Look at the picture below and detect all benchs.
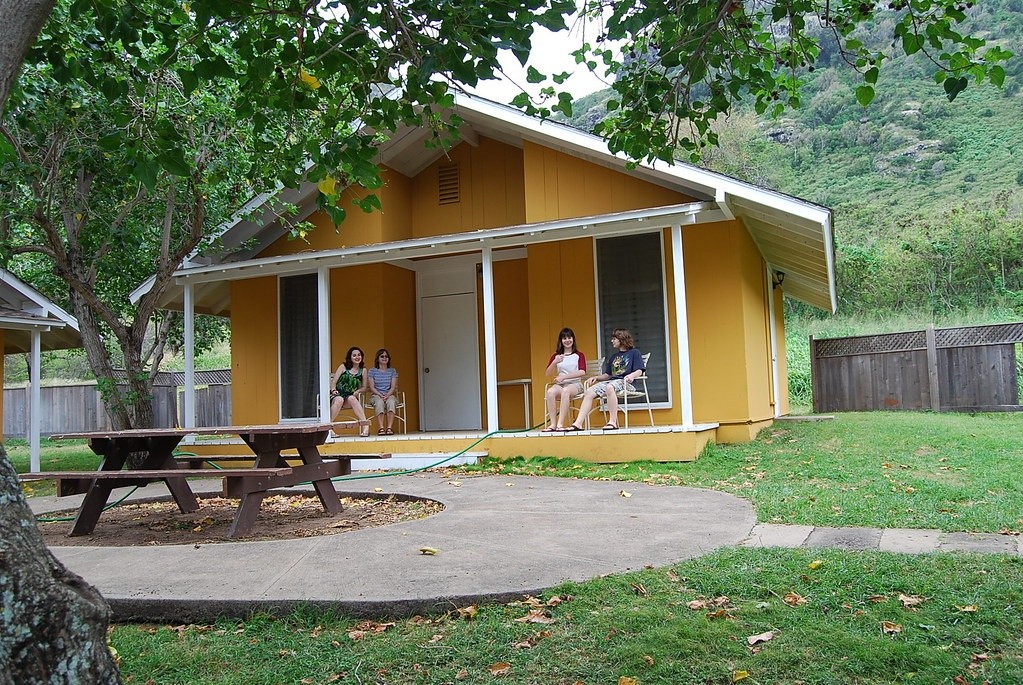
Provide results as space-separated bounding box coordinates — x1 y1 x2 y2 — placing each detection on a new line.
14 466 293 484
171 452 392 463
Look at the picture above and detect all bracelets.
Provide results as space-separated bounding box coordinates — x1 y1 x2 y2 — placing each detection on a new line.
332 390 335 393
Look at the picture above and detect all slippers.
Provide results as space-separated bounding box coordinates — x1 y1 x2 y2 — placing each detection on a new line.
360 432 369 436
555 427 564 432
331 433 339 438
565 425 584 431
542 428 555 432
603 424 619 430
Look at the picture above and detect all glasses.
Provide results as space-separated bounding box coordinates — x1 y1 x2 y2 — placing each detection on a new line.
380 355 389 358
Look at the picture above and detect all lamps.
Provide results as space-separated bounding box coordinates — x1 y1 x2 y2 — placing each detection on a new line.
772 271 785 289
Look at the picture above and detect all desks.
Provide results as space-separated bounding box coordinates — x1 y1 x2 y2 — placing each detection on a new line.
51 419 372 539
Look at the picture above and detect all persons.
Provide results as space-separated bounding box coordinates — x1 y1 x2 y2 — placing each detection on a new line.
368 349 398 436
542 327 586 431
564 328 646 431
330 347 370 438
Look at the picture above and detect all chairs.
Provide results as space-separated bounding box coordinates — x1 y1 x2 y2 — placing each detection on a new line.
585 351 652 429
364 371 407 435
316 373 363 436
543 355 606 430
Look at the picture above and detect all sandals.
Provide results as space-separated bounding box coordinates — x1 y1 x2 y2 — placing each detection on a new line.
386 428 393 435
378 429 385 435
